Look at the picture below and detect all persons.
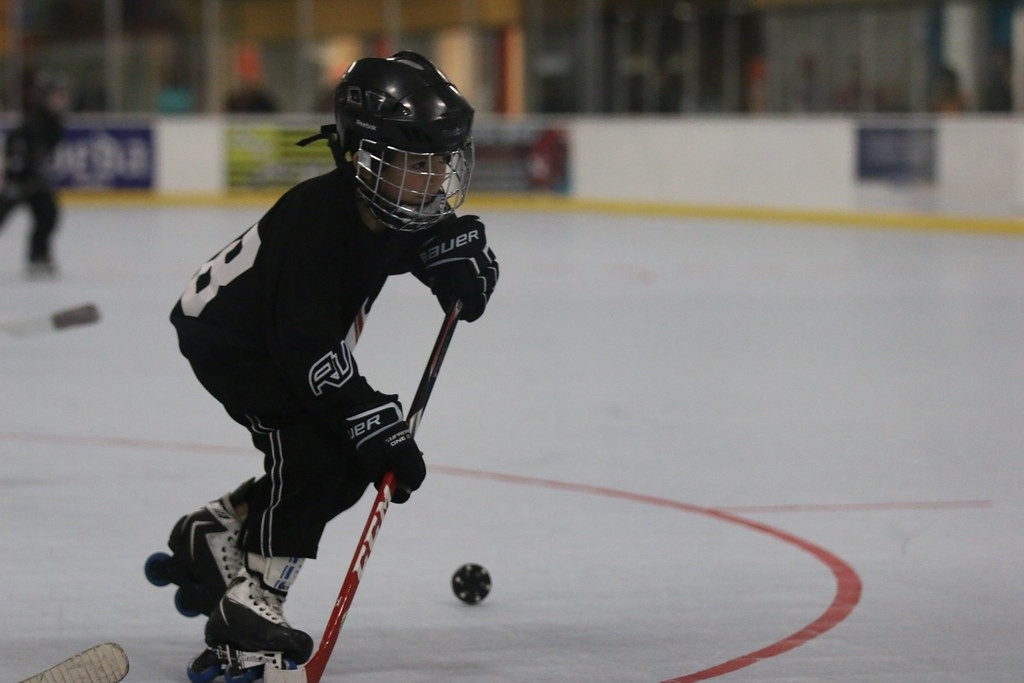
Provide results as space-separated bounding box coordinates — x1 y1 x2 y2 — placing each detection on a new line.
0 78 69 276
146 51 499 683
931 45 1014 113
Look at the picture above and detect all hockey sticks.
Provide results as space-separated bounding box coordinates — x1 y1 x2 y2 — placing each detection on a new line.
1 302 102 339
186 296 464 683
18 641 131 683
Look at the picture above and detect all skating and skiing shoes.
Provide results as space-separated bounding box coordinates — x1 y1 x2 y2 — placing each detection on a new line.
144 475 258 619
185 553 313 682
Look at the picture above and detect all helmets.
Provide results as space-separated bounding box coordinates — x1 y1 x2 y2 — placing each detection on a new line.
296 49 474 234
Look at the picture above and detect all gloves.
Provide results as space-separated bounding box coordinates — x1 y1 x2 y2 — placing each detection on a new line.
343 391 427 504
414 215 499 322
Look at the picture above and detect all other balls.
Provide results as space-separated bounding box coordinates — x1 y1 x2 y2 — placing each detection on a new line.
450 562 492 606
